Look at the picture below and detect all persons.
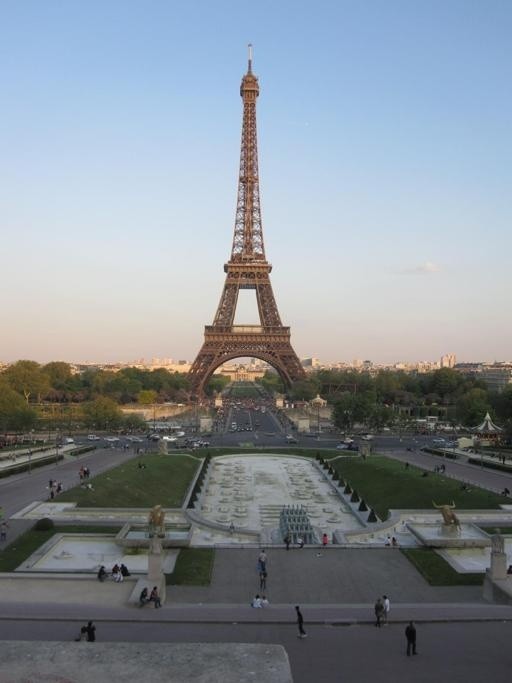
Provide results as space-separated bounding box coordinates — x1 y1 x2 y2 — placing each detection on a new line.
392 537 397 545
384 537 390 545
251 532 304 608
405 621 418 656
295 606 307 638
375 599 384 627
322 534 328 546
0 464 162 642
383 595 390 625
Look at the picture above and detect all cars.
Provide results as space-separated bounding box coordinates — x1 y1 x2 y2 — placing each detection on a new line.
87 430 211 449
287 435 299 444
336 434 360 451
